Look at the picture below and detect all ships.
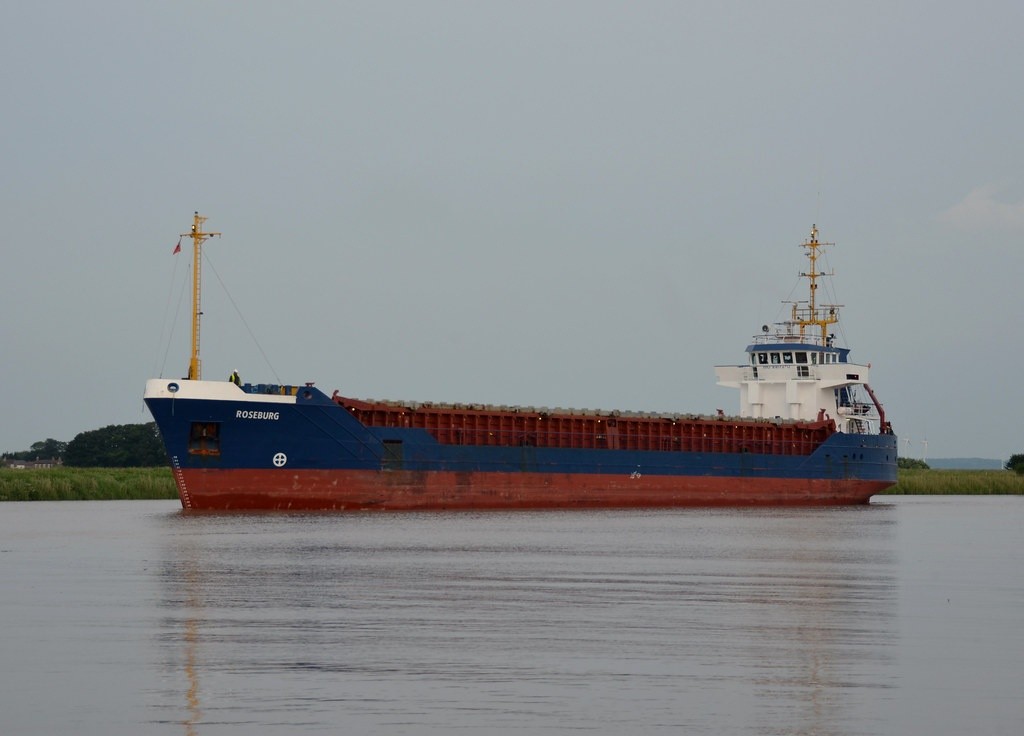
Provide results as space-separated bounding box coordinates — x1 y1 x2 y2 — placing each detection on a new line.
141 210 902 513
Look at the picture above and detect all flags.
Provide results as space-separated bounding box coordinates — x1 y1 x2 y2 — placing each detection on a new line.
172 241 181 255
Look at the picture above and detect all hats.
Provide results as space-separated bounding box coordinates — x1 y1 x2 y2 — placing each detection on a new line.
234 370 238 373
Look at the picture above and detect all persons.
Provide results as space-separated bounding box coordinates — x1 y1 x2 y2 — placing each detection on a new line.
229 369 241 387
